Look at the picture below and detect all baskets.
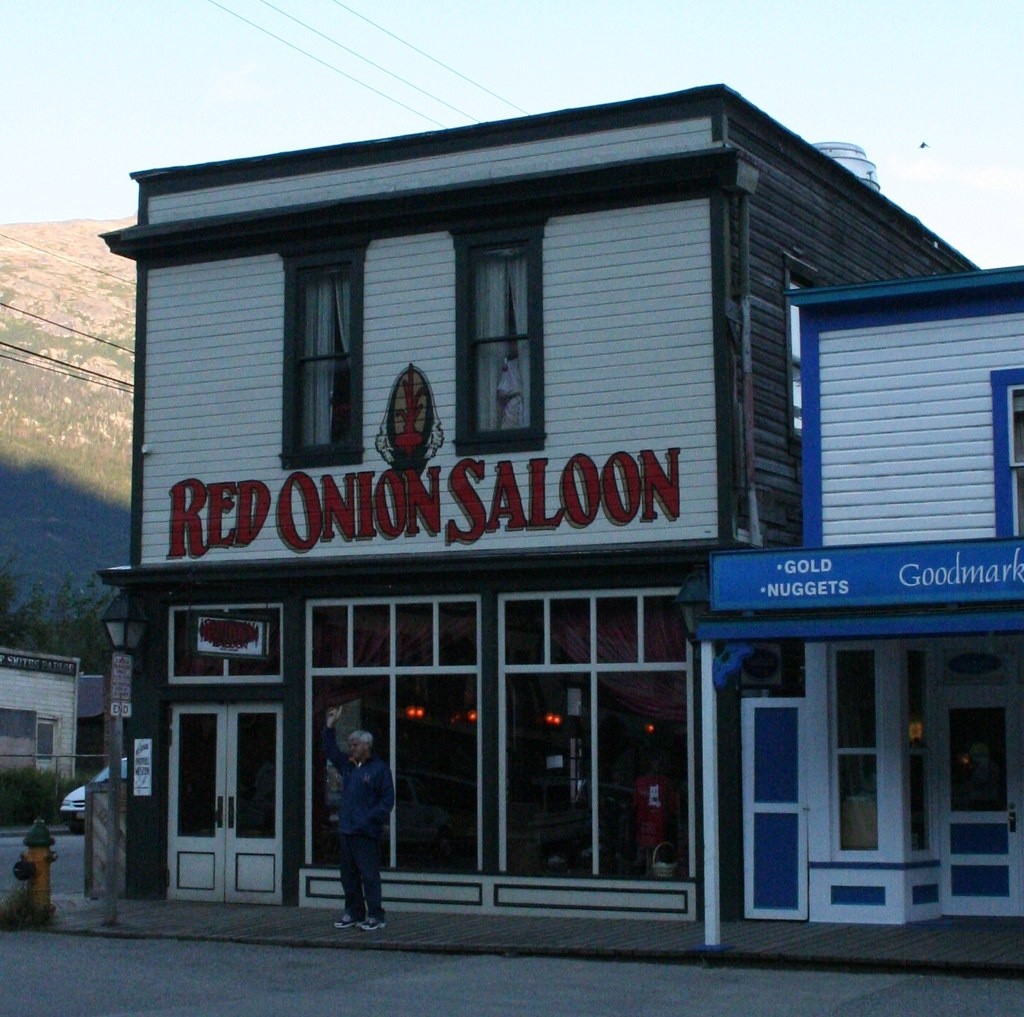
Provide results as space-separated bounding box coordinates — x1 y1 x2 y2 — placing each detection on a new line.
651 842 679 879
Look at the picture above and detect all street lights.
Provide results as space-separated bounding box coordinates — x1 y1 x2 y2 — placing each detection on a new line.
97 588 154 925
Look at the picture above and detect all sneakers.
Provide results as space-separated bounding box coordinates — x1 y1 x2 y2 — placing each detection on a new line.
360 917 385 930
334 914 368 928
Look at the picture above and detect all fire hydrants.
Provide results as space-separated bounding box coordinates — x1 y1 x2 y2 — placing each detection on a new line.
13 814 59 926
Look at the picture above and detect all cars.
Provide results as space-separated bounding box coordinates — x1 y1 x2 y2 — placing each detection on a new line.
58 756 128 835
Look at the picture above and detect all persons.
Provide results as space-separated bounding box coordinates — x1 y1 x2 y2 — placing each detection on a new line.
497 358 522 429
322 707 394 930
